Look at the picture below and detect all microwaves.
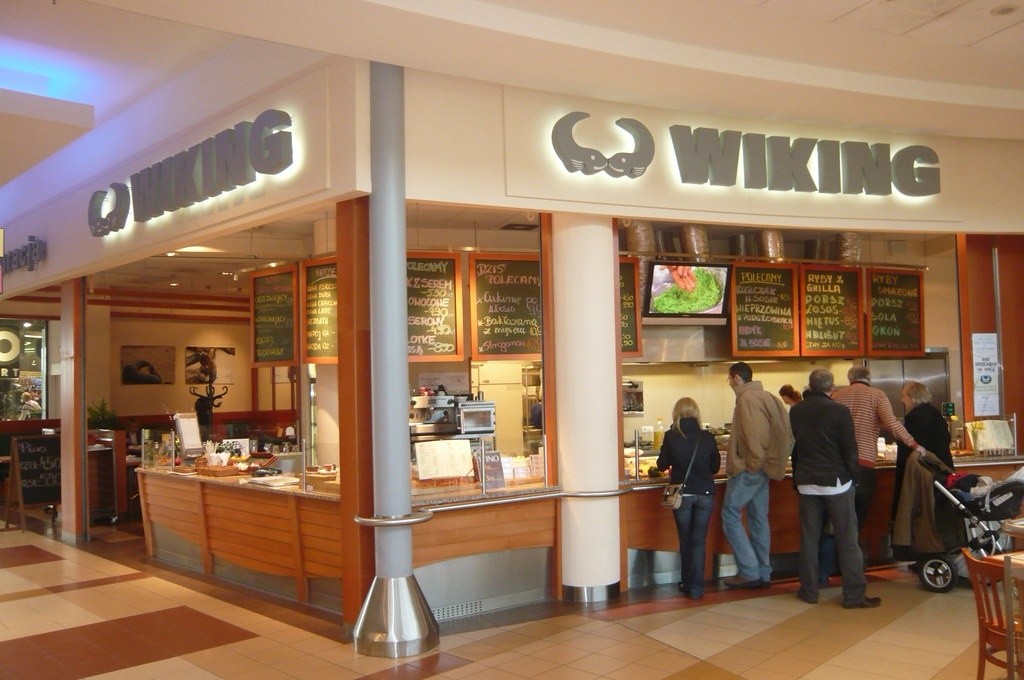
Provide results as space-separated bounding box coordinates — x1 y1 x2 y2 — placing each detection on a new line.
455 408 496 434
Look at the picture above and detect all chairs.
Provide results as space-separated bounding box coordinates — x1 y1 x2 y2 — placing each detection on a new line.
960 546 1024 680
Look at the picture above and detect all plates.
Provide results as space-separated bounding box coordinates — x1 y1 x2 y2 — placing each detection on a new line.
248 476 300 486
624 448 644 457
651 268 725 314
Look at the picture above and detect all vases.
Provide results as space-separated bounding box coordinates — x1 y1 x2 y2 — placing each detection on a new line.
969 431 981 456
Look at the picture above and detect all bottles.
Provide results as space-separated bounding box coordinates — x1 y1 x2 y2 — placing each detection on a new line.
216 441 242 457
264 442 298 453
154 442 160 459
437 384 446 396
163 438 177 458
252 443 256 452
654 417 663 451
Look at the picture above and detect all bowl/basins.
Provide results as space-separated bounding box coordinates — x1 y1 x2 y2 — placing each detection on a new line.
455 397 468 402
99 438 113 447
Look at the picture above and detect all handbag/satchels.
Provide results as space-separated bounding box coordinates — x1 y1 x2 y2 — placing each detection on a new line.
660 482 686 510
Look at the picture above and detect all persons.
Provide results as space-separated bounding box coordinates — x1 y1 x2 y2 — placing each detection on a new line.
529 393 543 455
656 397 721 600
818 365 926 587
797 369 882 609
125 417 143 457
894 379 956 571
780 383 802 406
660 265 697 293
722 362 793 588
19 390 41 420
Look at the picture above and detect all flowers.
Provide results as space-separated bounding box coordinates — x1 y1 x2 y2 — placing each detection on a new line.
950 414 984 446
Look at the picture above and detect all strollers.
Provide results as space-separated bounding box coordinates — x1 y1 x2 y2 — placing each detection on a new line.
911 448 1024 594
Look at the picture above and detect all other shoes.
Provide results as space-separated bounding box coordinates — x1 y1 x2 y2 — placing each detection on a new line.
679 581 699 599
845 595 881 607
725 574 771 588
798 589 816 604
908 562 917 568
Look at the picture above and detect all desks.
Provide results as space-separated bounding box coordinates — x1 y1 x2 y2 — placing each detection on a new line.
126 445 140 518
982 551 1024 619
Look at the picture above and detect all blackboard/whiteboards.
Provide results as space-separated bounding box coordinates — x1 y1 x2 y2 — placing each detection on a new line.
799 264 864 357
298 255 339 364
730 261 800 357
406 250 464 362
469 253 543 360
249 264 298 368
476 452 506 489
866 268 925 357
7 435 61 508
619 255 643 359
